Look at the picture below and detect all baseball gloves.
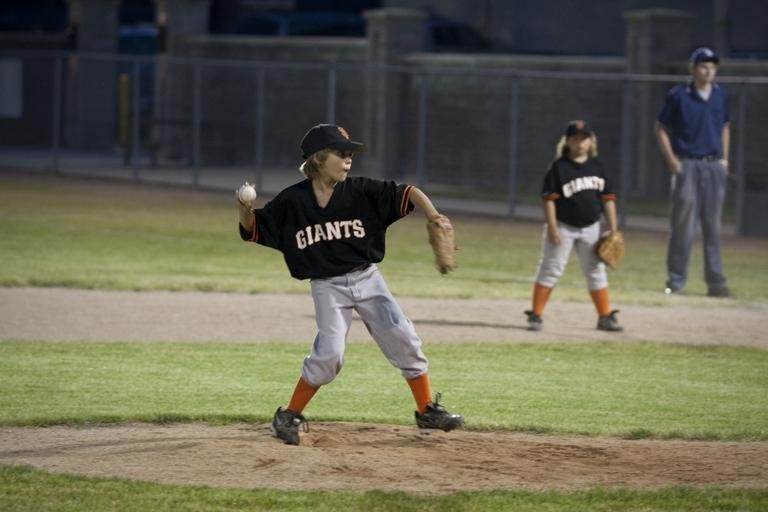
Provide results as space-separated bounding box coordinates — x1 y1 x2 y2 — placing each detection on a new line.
596 230 624 269
428 214 456 274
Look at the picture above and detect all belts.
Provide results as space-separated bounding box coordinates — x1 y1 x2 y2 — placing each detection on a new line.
683 155 717 160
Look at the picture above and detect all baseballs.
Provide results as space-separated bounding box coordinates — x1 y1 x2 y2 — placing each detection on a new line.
238 185 256 204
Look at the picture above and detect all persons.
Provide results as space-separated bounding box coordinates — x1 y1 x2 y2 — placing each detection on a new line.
231 121 465 447
651 46 737 299
521 118 630 333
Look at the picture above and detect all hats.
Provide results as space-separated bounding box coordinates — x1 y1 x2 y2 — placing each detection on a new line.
300 124 367 159
692 48 719 64
567 121 590 137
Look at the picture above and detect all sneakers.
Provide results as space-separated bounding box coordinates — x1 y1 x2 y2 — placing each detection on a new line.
707 285 731 297
597 309 623 331
526 310 542 329
274 407 305 445
665 283 682 295
414 401 464 430
